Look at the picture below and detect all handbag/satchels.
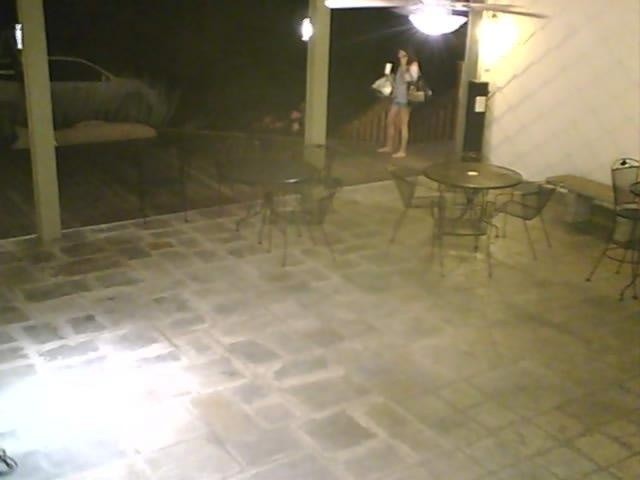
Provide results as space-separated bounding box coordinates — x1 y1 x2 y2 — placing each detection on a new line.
371 73 394 97
407 76 432 103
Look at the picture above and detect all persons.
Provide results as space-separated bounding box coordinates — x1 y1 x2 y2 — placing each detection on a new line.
377 48 420 158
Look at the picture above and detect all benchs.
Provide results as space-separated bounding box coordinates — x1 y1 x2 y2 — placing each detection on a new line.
546 174 640 242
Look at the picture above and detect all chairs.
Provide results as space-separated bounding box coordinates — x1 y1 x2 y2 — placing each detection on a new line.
221 143 343 268
389 160 557 278
139 135 190 225
584 158 640 303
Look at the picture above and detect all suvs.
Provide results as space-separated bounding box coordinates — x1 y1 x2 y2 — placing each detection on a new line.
1 56 169 125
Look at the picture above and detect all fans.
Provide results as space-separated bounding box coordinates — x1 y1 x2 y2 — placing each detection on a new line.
324 1 544 19
